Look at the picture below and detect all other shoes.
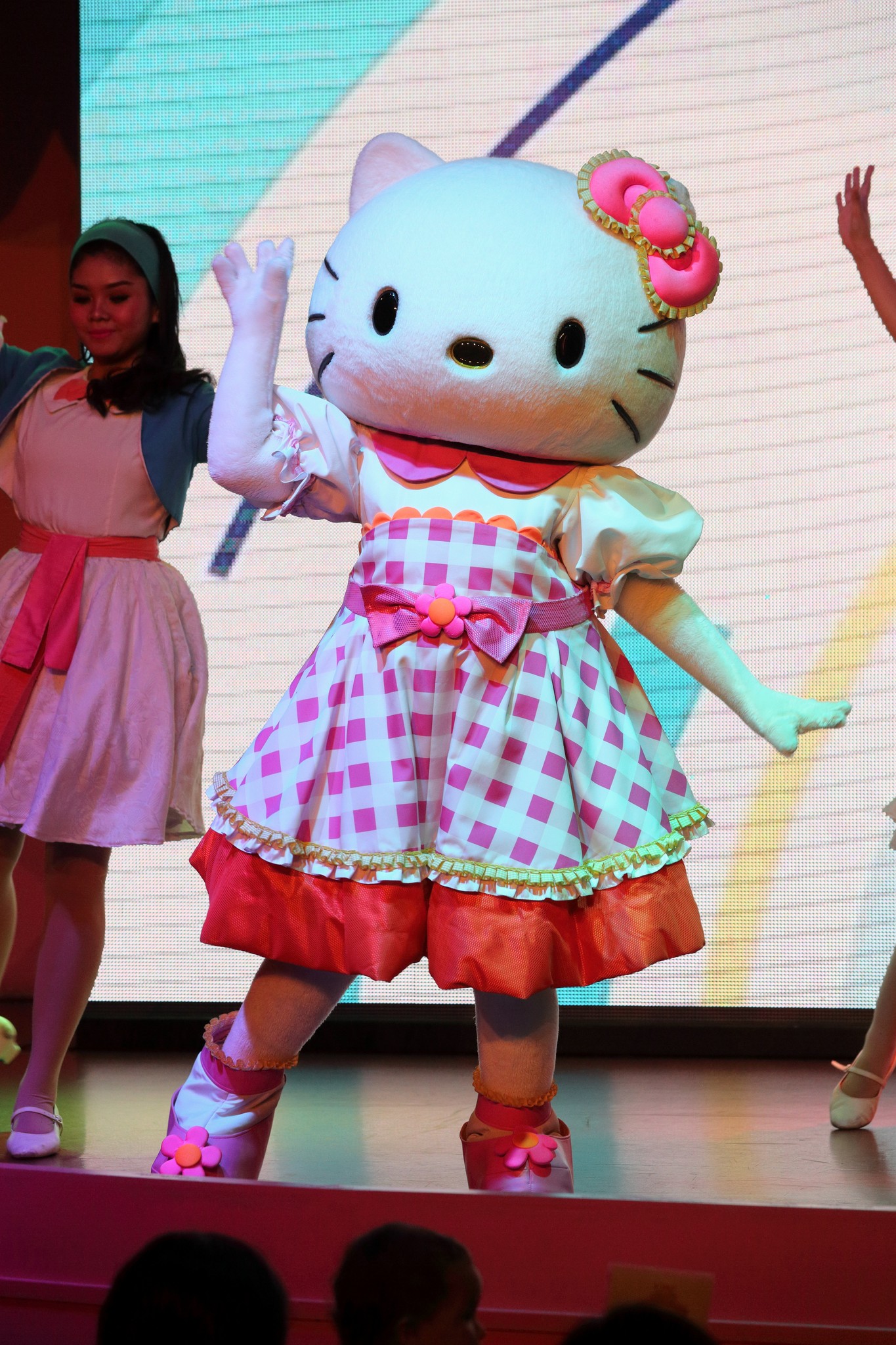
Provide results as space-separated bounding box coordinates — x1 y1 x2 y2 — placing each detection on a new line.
826 1048 896 1131
7 1101 64 1163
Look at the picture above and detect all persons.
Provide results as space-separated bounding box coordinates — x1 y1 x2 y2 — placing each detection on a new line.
566 1302 713 1345
330 1219 483 1345
0 219 215 1150
831 162 896 1131
92 1227 291 1345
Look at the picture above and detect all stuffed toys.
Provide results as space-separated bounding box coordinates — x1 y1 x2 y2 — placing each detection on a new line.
150 133 852 1199
